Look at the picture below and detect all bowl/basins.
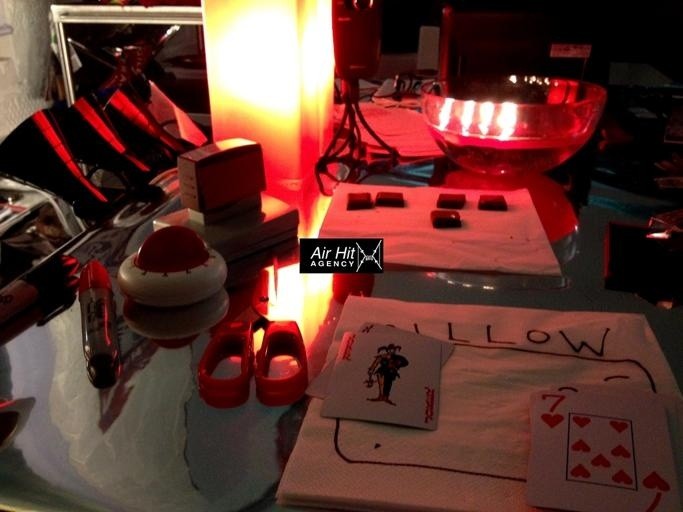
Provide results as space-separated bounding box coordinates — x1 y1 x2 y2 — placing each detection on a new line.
422 74 608 177
